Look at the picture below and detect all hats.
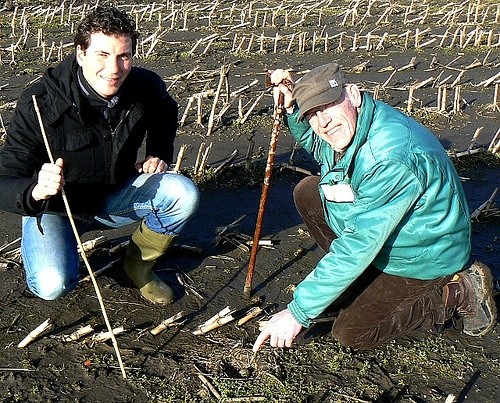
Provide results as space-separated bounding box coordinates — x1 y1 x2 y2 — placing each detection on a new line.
291 63 346 124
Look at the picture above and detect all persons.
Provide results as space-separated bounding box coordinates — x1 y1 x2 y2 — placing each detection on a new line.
252 64 498 353
0 6 200 307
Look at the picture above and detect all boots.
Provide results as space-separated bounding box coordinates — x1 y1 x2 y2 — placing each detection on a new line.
123 218 175 306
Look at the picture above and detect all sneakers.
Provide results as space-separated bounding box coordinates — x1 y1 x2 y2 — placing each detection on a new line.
438 261 497 336
